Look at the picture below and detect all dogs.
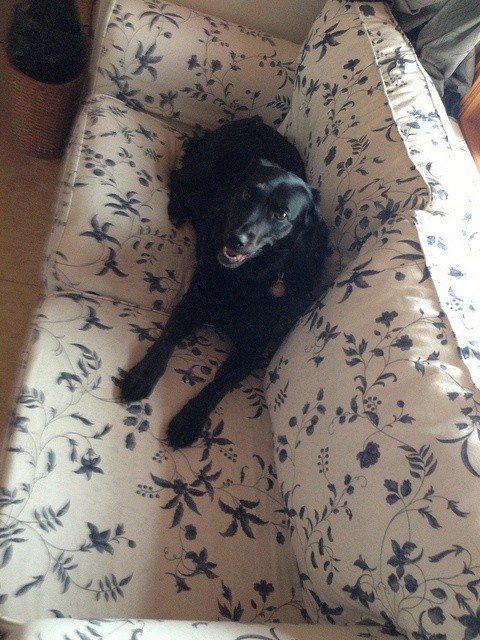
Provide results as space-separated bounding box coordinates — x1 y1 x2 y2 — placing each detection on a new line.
120 118 329 450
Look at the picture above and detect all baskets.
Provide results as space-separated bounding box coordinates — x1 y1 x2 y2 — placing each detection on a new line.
8 57 85 160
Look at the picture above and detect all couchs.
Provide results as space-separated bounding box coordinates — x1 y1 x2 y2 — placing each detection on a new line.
0 1 479 640
